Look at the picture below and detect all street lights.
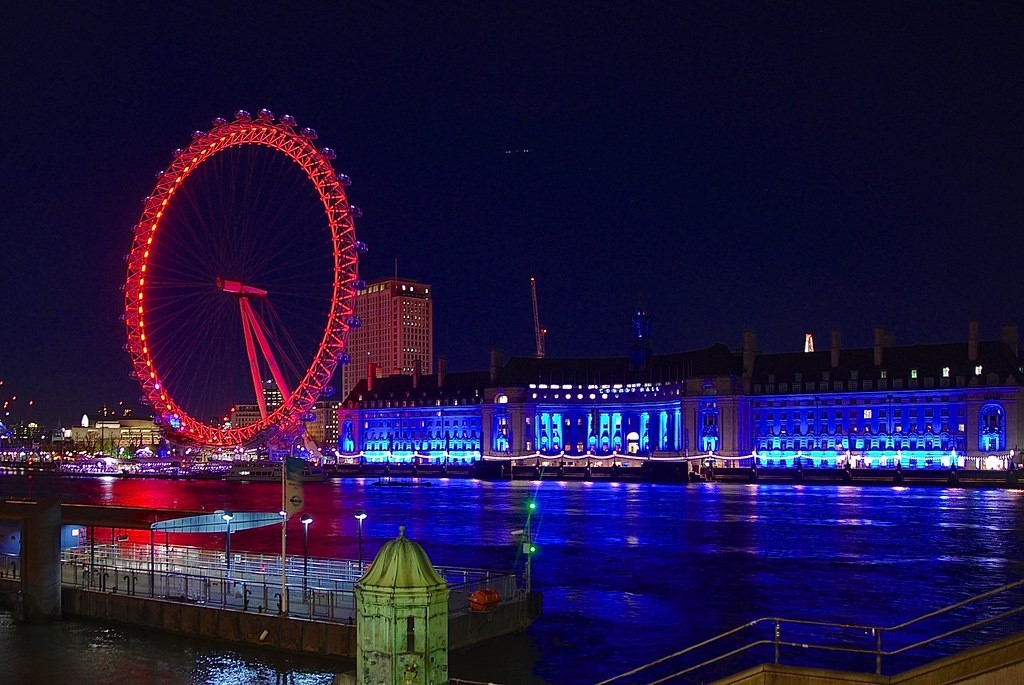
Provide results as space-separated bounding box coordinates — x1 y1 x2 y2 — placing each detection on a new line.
536 451 540 466
586 451 591 473
612 451 618 467
560 450 564 468
355 511 367 573
222 513 233 593
301 514 312 600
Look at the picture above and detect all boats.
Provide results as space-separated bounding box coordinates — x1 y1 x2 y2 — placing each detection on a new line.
221 460 326 482
373 475 431 486
